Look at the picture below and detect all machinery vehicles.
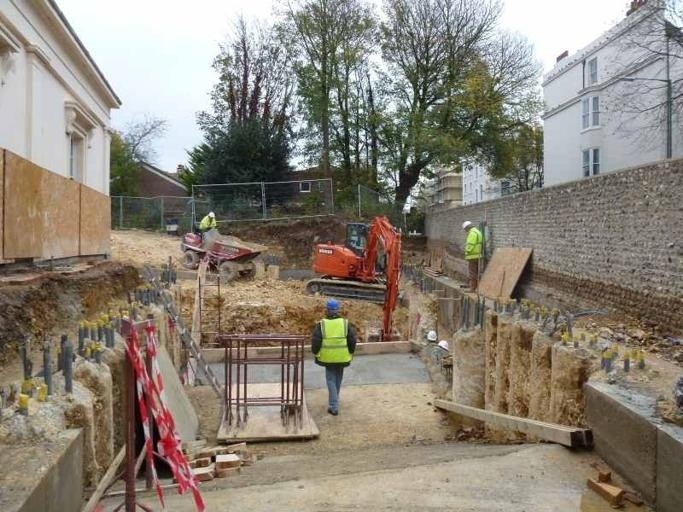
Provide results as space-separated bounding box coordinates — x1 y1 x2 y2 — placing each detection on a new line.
304 215 403 342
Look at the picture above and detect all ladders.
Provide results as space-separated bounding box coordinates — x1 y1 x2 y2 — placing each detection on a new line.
149 277 225 398
199 276 220 348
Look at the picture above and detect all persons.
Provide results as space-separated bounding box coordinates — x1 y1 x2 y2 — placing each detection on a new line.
456 220 486 295
311 296 356 416
196 212 218 232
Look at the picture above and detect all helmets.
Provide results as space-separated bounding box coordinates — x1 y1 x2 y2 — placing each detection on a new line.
462 220 471 230
326 300 338 310
209 211 215 218
438 340 449 351
427 330 437 341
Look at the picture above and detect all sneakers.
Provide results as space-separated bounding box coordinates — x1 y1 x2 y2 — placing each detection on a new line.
328 408 338 416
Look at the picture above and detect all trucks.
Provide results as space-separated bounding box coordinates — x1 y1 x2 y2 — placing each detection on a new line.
181 230 268 282
